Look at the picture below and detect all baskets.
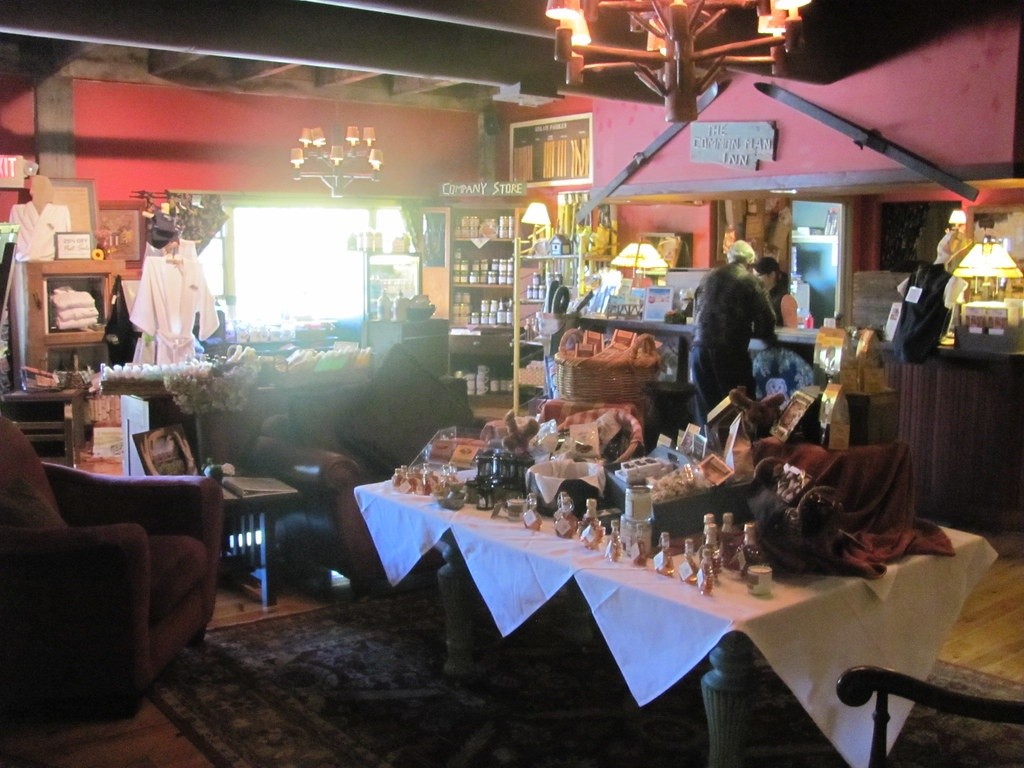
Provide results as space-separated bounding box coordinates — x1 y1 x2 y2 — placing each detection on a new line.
555 352 656 404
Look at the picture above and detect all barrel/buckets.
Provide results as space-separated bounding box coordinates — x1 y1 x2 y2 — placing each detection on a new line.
530 462 601 520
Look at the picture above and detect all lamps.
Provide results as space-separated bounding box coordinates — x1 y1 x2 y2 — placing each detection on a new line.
545 0 812 123
520 203 551 258
610 242 669 289
952 244 1024 335
290 99 384 197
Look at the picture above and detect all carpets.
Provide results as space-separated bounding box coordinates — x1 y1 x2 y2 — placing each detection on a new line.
141 599 1024 768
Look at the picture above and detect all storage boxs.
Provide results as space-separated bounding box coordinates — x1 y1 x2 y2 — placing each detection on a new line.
554 327 660 404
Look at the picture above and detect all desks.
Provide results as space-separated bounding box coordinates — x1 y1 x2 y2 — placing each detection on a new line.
219 476 302 607
353 479 1000 768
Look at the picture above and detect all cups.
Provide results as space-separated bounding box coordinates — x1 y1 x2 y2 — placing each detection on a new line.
462 365 489 396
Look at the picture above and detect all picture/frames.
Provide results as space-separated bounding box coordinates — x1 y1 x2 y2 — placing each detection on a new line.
53 232 94 260
47 177 97 258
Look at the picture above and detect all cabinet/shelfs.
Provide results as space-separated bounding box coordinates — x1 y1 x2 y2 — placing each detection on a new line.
443 201 521 417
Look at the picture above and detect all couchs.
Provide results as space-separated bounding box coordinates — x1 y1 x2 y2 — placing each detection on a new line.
0 414 225 716
251 353 488 598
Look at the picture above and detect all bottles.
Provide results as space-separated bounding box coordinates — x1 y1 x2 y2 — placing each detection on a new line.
655 531 674 575
204 346 255 376
100 362 213 385
679 513 772 598
622 487 652 567
466 454 536 511
525 493 542 531
490 377 514 395
527 271 562 300
376 290 391 319
366 232 383 256
578 498 623 563
452 291 514 326
259 350 275 382
790 280 814 332
392 461 459 496
455 215 515 239
525 312 543 342
554 492 577 540
453 258 514 284
394 291 411 319
288 346 371 371
824 207 839 235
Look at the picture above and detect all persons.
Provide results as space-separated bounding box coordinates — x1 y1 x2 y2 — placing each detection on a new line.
687 239 777 413
752 257 788 328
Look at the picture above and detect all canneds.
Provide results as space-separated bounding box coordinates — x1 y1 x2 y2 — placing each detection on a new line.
453 216 516 326
526 272 563 300
